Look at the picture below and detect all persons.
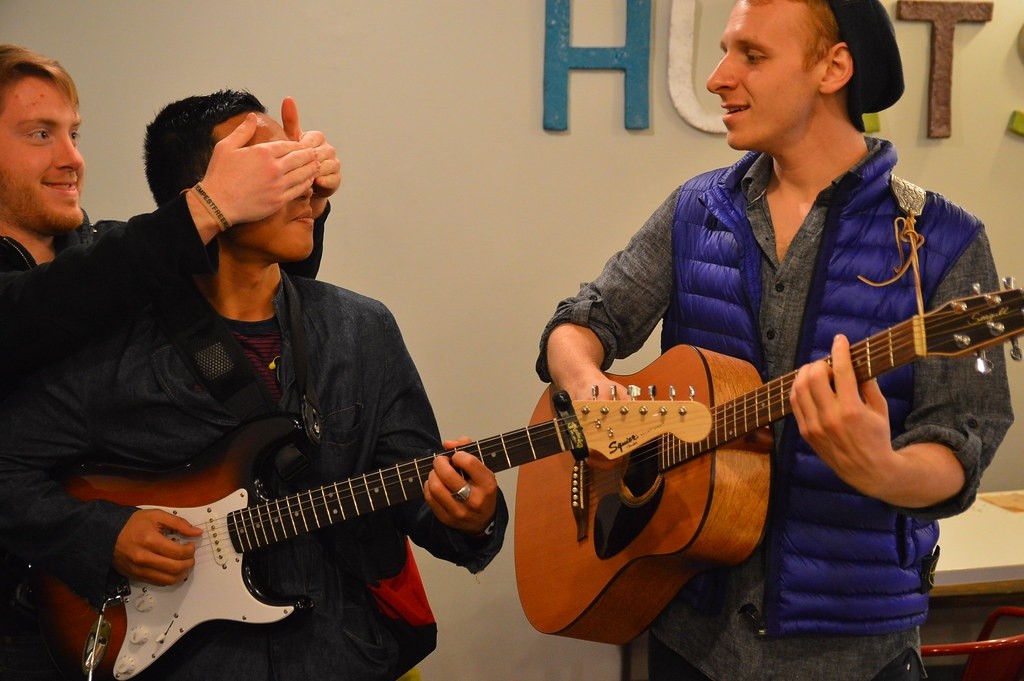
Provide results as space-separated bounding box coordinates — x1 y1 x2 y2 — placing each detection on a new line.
0 47 340 382
534 0 1013 681
0 91 509 681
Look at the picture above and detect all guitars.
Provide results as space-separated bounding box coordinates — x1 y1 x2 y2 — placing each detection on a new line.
34 385 713 681
514 276 1024 646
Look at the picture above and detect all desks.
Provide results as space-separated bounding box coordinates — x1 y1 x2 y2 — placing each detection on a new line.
928 490 1024 596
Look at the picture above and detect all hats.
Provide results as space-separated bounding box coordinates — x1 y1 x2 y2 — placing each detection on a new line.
830 0 904 132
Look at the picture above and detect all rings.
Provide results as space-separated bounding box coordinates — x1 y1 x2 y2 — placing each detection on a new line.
453 484 472 501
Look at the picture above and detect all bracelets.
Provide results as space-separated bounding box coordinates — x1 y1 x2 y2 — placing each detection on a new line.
191 181 232 233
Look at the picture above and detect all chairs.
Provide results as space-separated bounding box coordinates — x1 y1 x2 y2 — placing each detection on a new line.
920 606 1024 681
